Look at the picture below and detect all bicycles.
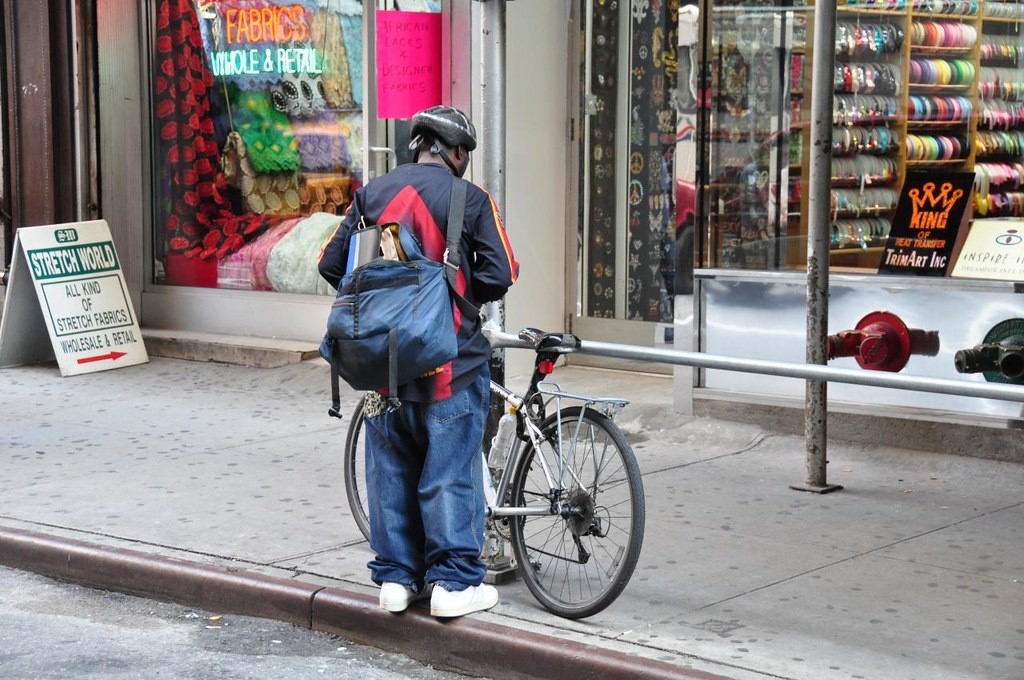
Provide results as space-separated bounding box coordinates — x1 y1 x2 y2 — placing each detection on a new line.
343 327 646 620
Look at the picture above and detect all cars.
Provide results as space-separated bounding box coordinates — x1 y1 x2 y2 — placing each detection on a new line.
675 124 804 294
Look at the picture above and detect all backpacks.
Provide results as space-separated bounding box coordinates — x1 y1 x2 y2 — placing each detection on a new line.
316 175 482 420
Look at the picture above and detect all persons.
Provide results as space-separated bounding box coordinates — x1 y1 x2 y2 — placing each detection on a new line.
318 106 520 619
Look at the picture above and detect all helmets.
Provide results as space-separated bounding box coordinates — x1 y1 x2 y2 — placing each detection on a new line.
408 103 478 177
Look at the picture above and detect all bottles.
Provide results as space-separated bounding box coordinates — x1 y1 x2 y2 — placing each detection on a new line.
488 405 517 470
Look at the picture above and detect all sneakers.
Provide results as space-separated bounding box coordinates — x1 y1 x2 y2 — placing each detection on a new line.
380 581 433 612
430 583 499 617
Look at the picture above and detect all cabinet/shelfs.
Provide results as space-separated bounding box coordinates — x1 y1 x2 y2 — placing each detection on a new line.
708 1 1024 267
